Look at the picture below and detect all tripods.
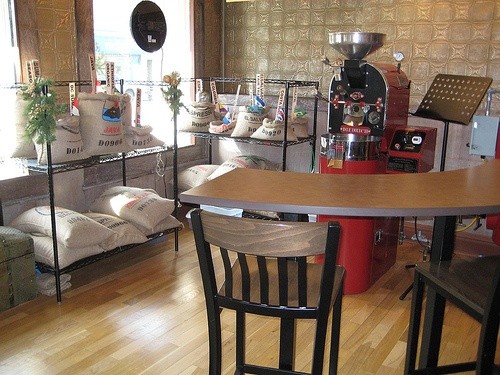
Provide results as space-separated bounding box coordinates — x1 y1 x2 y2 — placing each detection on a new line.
399 74 493 302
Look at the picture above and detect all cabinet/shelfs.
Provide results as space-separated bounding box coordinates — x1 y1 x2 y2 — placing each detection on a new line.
183 77 319 173
6 80 182 303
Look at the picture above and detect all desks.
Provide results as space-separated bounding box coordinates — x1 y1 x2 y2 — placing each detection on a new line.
179 157 500 375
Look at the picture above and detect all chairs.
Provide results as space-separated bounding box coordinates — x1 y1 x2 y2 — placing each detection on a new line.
403 254 500 375
190 208 347 375
